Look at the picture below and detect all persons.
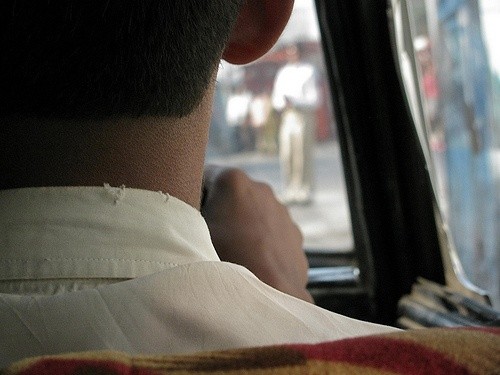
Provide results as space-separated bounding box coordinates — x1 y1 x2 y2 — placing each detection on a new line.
0 0 411 375
271 40 326 207
